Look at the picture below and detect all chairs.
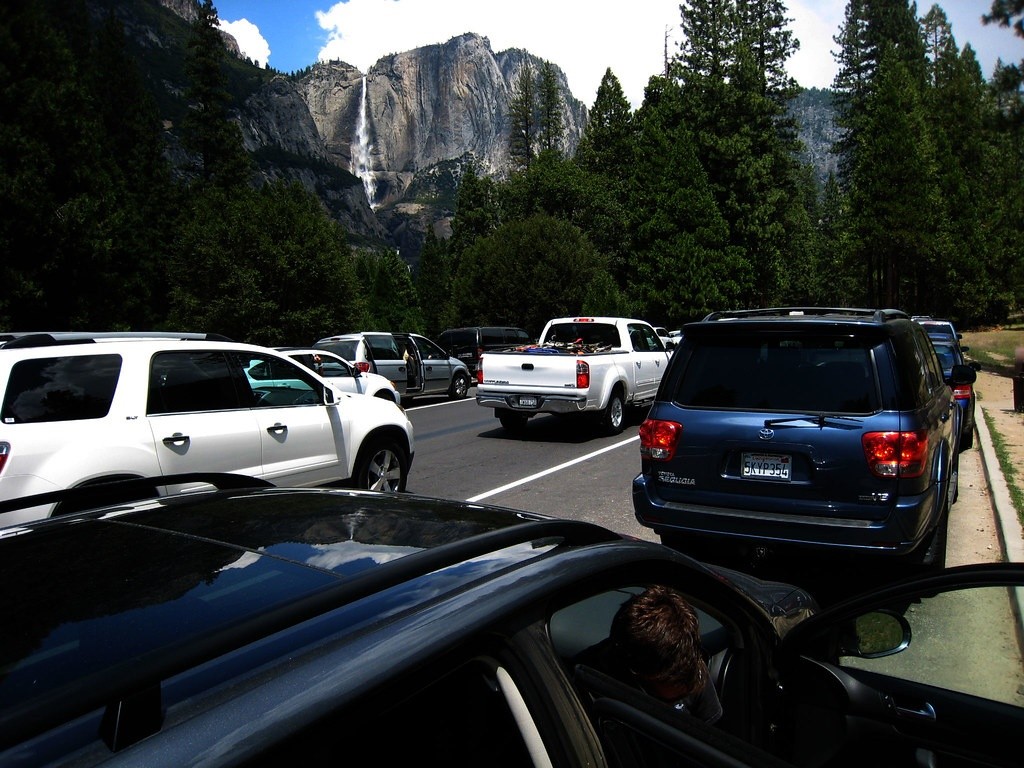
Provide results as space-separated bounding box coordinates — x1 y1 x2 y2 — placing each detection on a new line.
818 358 875 410
164 368 199 409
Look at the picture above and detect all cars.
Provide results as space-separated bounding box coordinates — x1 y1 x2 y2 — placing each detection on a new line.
909 315 980 446
633 305 977 568
0 330 416 542
653 326 674 350
0 472 1024 768
668 331 681 339
244 346 402 406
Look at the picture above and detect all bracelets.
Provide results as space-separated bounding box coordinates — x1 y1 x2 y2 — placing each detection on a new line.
318 364 323 367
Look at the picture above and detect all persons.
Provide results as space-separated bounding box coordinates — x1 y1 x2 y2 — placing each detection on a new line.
309 354 324 375
573 586 722 726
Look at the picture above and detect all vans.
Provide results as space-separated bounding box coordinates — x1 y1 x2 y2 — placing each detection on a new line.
311 332 472 401
436 326 534 376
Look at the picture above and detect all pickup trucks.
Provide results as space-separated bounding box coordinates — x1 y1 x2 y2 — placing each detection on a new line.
476 315 674 430
